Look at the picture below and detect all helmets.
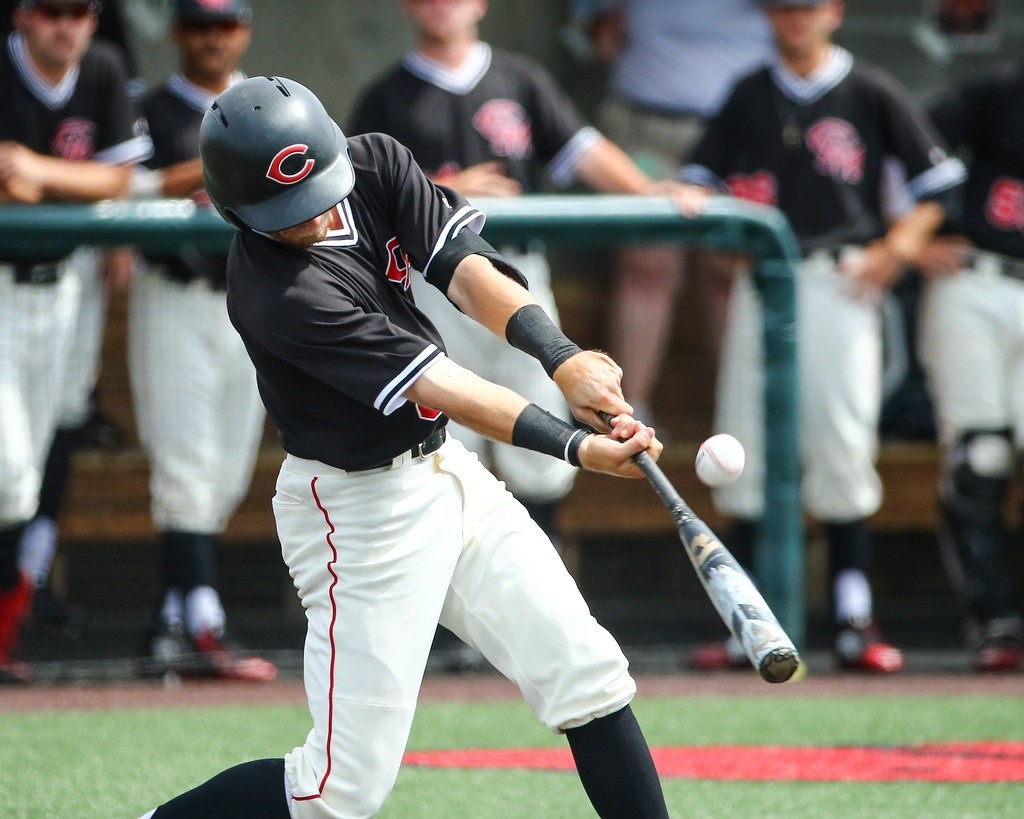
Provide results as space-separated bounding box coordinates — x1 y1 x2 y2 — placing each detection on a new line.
199 75 355 233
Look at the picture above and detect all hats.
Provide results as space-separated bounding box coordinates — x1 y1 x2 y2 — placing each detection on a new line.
177 0 250 27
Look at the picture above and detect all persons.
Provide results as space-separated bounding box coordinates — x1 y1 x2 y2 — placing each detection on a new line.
0 0 155 676
670 2 972 676
140 74 674 819
554 0 1024 675
114 0 281 685
342 1 702 675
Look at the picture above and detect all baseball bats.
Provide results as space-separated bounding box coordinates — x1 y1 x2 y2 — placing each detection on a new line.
599 413 801 684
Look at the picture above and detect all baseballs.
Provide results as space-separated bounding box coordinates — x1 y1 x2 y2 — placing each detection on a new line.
694 433 745 488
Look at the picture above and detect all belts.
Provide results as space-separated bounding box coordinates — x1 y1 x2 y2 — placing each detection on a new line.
13 262 61 286
349 425 448 473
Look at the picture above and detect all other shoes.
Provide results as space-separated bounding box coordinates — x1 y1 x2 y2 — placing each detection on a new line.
971 636 1020 669
172 640 279 683
838 627 906 672
688 640 755 669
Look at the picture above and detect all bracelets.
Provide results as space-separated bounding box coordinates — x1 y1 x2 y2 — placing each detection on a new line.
505 304 583 382
512 402 590 470
